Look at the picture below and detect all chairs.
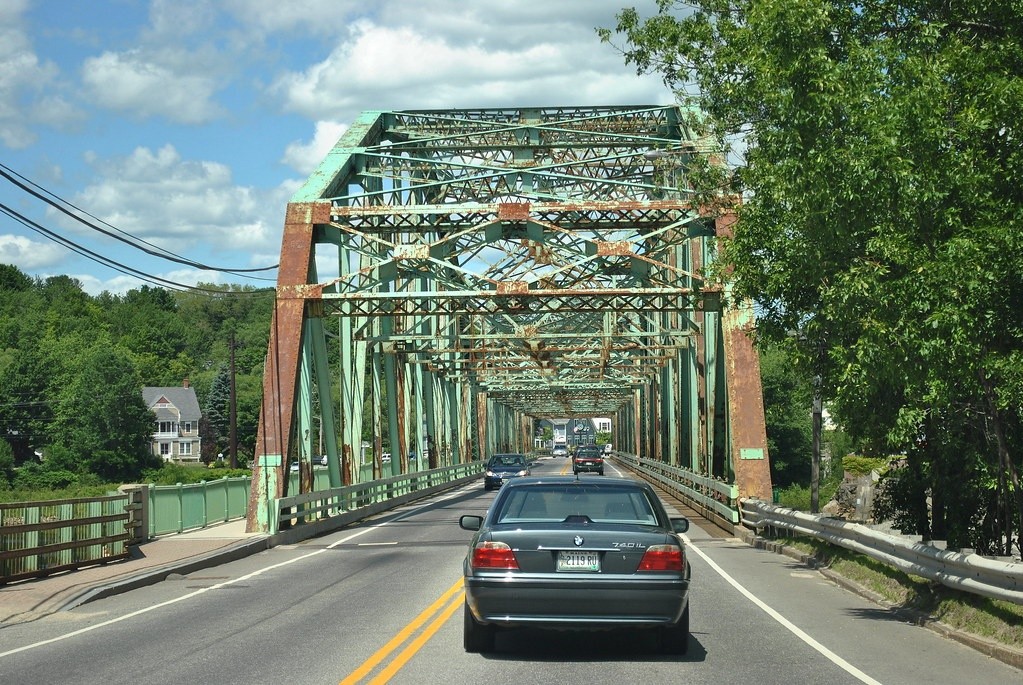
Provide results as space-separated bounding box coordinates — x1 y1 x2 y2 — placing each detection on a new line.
516 502 546 518
606 502 639 518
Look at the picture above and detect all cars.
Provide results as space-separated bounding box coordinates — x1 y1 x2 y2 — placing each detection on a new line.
482 454 533 491
291 461 299 471
571 443 612 476
457 473 693 654
551 443 570 458
